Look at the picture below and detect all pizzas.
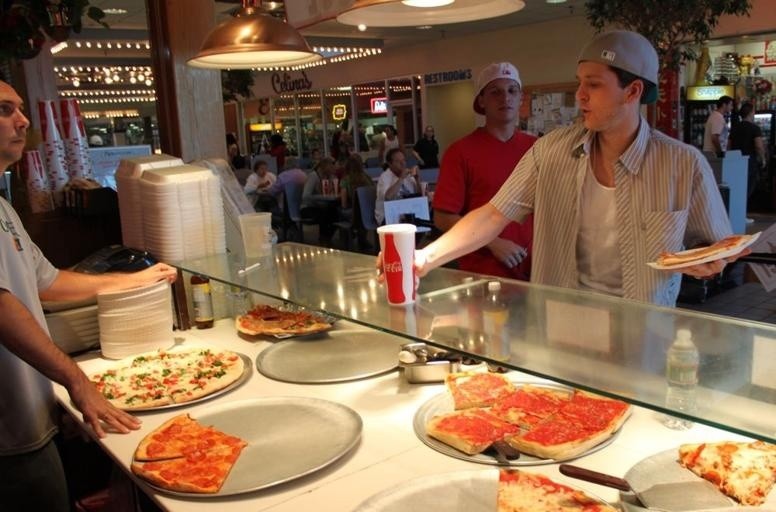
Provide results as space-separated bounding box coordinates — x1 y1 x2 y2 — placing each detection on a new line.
235 304 332 336
88 348 244 410
427 371 630 462
657 235 750 266
497 467 616 512
130 412 247 493
678 440 776 506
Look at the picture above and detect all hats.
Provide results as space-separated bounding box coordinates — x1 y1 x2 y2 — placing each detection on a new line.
472 63 522 115
577 31 658 104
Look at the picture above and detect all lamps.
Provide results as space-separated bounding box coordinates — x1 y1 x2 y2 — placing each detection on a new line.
336 1 526 31
182 0 323 72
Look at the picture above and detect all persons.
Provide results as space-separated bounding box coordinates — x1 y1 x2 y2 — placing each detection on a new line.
431 61 542 282
702 95 732 152
375 29 753 375
728 103 768 223
224 124 444 249
0 80 178 511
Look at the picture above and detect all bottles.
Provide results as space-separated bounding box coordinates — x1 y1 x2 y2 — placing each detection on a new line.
229 261 249 319
456 274 483 338
483 279 513 372
400 184 406 198
693 105 717 118
751 95 776 111
692 133 703 147
662 330 702 431
692 120 704 131
189 260 216 330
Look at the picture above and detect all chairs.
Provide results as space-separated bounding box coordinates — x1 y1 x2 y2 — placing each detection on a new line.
234 147 440 244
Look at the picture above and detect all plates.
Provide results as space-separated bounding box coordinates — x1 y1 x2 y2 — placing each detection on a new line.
46 305 99 355
98 275 174 360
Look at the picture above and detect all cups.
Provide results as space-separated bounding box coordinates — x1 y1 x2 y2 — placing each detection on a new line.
376 223 418 309
322 180 329 195
22 149 52 216
417 182 428 196
39 99 70 208
333 179 339 193
239 210 272 259
57 97 94 180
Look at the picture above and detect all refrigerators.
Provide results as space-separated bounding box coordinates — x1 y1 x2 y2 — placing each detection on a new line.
684 86 745 161
